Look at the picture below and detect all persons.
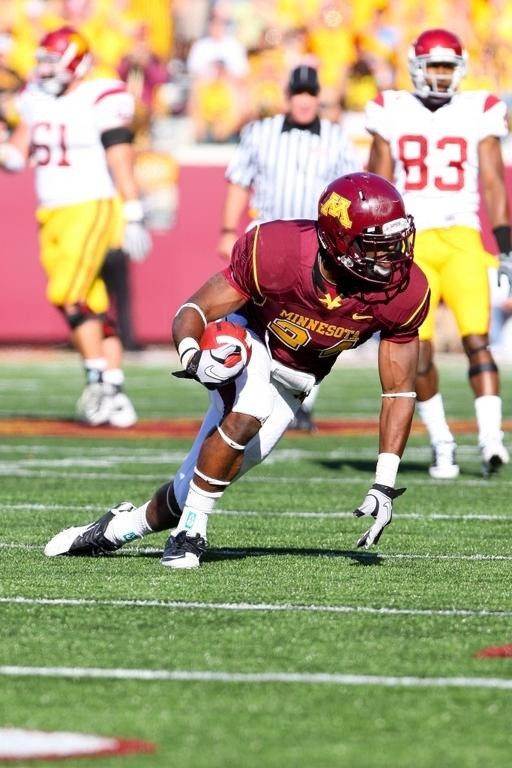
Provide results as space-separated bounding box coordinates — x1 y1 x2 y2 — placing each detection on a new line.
362 28 512 480
220 66 360 432
0 1 512 144
0 25 143 429
43 172 431 568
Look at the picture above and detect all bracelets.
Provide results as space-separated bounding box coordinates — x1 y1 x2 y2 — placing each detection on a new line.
220 227 237 234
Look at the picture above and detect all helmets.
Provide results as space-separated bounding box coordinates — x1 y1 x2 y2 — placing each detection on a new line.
317 172 416 285
33 27 93 96
409 29 468 106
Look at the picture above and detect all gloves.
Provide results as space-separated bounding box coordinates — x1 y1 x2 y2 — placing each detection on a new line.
353 484 407 550
121 221 151 261
498 252 512 296
171 343 246 391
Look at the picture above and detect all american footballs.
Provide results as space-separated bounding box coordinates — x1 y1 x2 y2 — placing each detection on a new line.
201 322 252 369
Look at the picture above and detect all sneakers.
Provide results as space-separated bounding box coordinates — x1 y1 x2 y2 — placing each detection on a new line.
429 441 459 479
479 431 508 468
77 368 138 427
43 502 137 556
161 529 209 568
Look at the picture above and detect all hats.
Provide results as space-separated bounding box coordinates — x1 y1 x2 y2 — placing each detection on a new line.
289 67 317 94
